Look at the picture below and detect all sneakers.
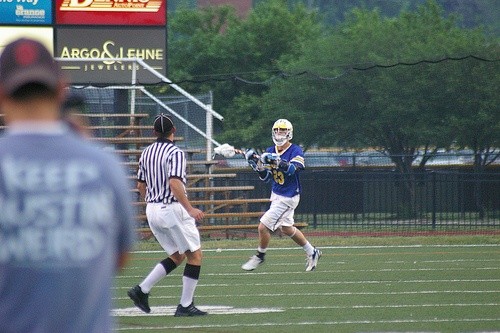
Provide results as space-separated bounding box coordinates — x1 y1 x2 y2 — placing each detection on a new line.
175 303 207 317
128 285 151 313
305 246 323 272
242 255 264 270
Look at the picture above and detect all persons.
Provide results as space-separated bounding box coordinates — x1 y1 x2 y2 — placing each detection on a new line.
1 38 139 333
127 112 208 316
240 118 323 271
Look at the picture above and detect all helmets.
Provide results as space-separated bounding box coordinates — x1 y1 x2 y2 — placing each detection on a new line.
272 119 293 146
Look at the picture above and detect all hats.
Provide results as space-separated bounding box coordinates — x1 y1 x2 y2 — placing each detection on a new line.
152 113 173 133
0 39 57 95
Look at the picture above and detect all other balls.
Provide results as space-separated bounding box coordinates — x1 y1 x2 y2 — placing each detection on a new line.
216 249 221 252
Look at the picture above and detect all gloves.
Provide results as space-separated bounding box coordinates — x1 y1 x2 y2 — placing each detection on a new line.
260 153 280 165
244 147 259 168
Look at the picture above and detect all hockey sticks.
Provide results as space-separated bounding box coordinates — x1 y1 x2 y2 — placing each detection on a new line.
213 143 284 166
